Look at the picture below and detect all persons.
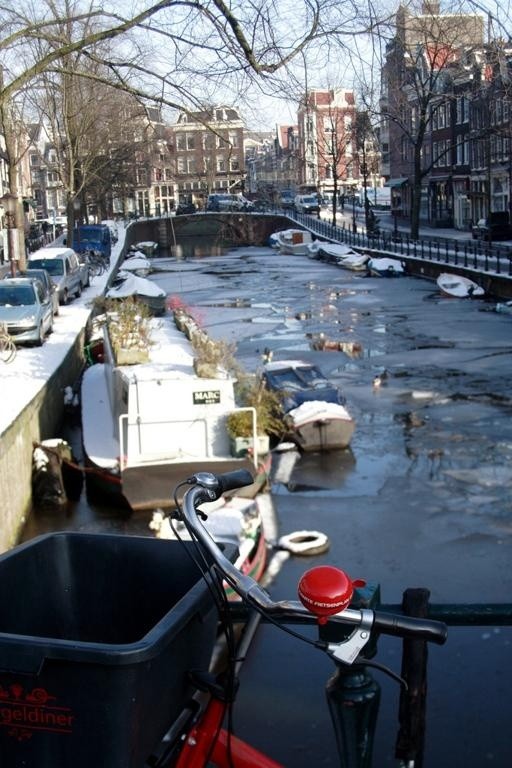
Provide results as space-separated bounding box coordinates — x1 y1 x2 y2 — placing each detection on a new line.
42 222 48 237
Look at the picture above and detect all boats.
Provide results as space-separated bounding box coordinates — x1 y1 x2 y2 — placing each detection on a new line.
259 356 358 453
270 226 312 256
108 241 170 316
305 238 406 277
79 306 275 512
436 271 485 298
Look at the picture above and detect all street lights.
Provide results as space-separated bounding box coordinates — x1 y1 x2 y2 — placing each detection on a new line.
1 193 24 278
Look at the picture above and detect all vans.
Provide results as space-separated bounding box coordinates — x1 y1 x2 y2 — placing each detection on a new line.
207 193 256 212
24 247 80 306
72 223 112 258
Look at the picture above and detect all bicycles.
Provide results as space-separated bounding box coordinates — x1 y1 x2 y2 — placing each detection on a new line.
1 467 448 768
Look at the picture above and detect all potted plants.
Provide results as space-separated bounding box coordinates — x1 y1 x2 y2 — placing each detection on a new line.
226 402 270 459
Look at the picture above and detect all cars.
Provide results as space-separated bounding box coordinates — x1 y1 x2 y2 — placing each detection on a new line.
73 251 93 292
470 210 511 242
278 189 295 206
1 276 55 347
102 219 119 241
3 267 60 316
293 194 320 214
